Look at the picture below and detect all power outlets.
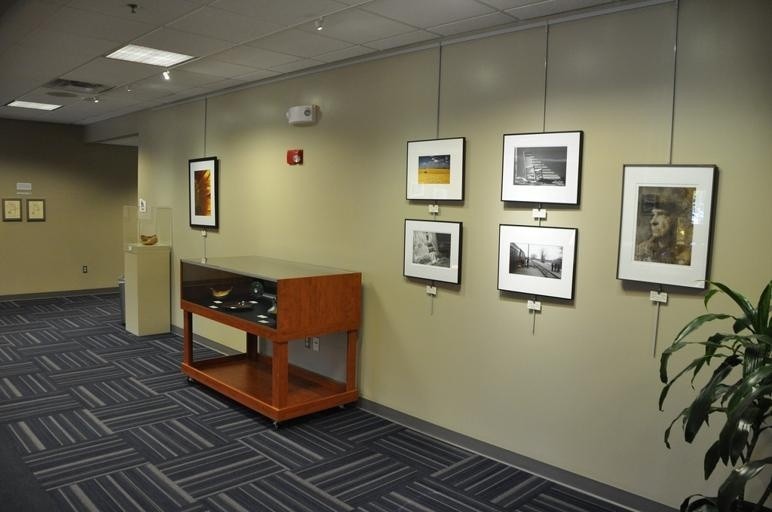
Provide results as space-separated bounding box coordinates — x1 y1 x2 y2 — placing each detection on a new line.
83 265 87 273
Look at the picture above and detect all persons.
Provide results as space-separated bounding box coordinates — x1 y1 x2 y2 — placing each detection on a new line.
632 195 687 264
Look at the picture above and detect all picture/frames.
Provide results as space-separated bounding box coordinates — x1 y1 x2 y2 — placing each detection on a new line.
188 156 218 228
497 224 578 300
616 162 719 290
405 137 465 202
403 219 461 285
3 198 22 222
26 198 46 222
501 130 583 205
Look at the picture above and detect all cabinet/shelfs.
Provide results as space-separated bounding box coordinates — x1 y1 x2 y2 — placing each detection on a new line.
180 257 362 431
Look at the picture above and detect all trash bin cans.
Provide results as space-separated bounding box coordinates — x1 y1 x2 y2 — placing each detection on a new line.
117 275 125 325
